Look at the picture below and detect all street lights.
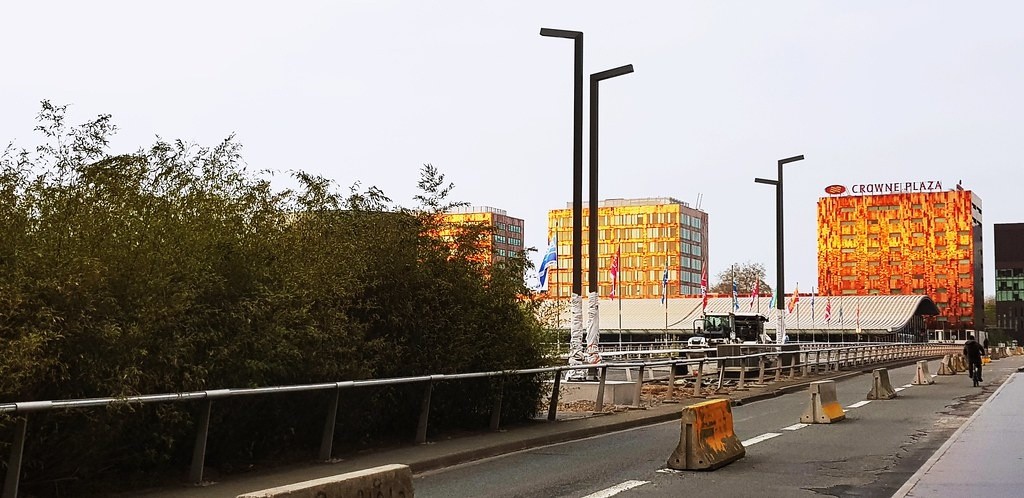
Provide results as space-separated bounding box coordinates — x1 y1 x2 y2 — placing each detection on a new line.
540 28 633 382
754 155 804 352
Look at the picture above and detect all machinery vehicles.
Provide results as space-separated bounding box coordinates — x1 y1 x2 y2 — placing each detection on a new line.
688 311 767 357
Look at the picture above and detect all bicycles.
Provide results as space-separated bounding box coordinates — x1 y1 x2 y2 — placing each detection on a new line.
972 363 979 387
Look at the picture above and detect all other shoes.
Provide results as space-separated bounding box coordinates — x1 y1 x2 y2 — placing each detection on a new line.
978 378 983 382
969 374 972 378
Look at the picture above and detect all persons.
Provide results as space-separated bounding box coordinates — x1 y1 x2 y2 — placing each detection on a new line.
963 335 985 382
983 337 989 349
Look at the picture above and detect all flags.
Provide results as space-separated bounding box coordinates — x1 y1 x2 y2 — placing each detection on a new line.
768 283 778 315
660 250 668 304
534 233 557 292
732 270 740 311
700 259 708 313
823 294 831 324
748 274 759 311
811 293 814 324
837 298 843 322
609 243 620 300
786 285 799 313
855 301 859 327
